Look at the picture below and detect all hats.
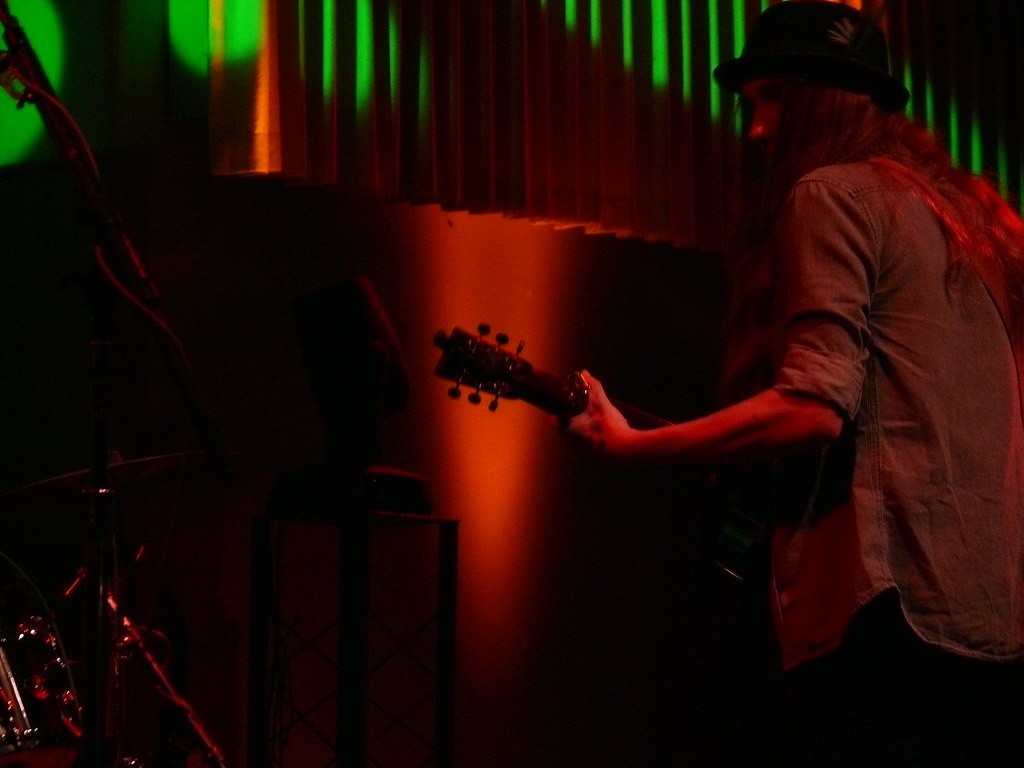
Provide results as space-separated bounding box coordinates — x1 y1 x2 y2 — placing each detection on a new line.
714 1 910 112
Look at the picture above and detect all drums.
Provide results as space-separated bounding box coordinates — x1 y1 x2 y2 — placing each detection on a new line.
0 607 83 768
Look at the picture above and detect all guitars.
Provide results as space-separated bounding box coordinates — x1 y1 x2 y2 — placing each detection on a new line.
431 322 770 588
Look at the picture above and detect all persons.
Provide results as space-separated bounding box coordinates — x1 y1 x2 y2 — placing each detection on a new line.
551 0 1024 768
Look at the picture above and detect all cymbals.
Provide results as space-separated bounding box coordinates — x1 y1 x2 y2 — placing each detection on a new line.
21 445 214 492
